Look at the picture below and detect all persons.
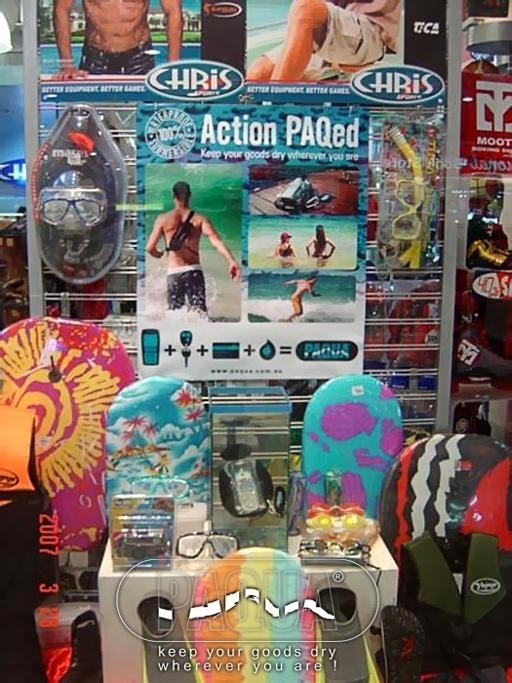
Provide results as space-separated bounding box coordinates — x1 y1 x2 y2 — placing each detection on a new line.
144 181 242 319
49 0 186 79
270 231 296 269
303 223 336 260
283 275 322 321
242 0 403 81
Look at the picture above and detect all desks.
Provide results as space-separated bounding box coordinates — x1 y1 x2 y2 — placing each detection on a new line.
97 502 400 682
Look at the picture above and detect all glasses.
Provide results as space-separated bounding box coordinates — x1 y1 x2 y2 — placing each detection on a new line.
39 196 103 226
393 178 425 242
176 532 240 561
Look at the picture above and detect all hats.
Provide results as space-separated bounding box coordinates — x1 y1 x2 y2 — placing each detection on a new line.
280 233 292 244
